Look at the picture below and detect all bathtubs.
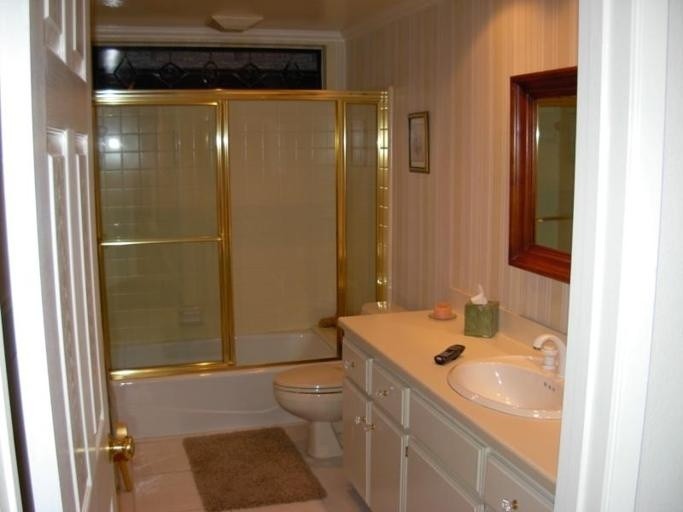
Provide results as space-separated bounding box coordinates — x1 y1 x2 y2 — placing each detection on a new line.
110 326 338 439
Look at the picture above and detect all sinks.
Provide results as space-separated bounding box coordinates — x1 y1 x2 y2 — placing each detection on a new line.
447 355 563 420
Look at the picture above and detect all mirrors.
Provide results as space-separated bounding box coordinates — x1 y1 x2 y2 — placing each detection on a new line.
506 62 577 286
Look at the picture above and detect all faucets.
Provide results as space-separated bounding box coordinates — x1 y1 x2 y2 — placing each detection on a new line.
532 332 567 378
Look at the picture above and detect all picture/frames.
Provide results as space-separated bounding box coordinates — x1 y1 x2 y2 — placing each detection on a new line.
407 111 430 177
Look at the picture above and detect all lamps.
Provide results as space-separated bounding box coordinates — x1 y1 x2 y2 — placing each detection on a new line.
211 12 263 32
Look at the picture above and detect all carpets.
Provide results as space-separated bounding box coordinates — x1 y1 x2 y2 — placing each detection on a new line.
183 423 327 511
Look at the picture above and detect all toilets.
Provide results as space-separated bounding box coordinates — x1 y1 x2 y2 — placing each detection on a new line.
273 301 409 459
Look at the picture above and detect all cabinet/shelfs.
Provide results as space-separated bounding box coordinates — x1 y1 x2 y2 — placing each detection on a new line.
404 389 554 511
340 338 407 511
